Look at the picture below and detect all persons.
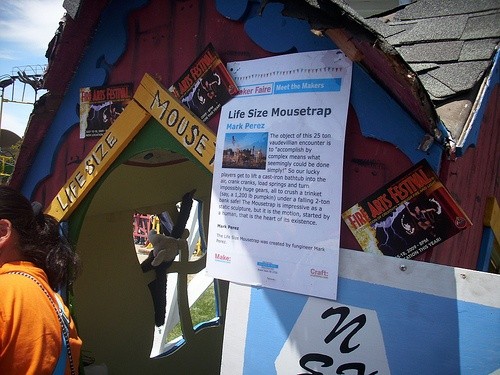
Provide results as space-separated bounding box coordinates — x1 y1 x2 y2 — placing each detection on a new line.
0 182 82 375
405 203 437 237
201 79 218 101
109 102 121 125
